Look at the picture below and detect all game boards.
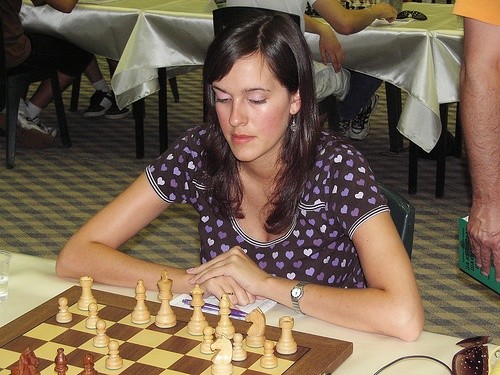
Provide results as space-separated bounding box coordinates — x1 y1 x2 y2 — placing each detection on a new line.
0 285 353 374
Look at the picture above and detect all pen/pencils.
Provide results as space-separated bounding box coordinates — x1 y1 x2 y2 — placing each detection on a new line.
181 299 249 317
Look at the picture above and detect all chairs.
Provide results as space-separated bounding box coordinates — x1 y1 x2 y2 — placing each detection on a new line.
0 20 71 169
377 182 416 262
212 6 339 134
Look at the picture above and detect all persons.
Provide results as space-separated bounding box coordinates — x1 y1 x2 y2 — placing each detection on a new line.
0 0 131 148
451 0 500 284
226 0 401 140
56 13 424 341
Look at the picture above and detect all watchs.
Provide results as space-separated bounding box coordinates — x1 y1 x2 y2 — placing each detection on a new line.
290 281 309 315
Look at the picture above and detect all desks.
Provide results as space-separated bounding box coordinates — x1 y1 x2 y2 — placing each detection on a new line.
0 253 500 375
17 0 466 199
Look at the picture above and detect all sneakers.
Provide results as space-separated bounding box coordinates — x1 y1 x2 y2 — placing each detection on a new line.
334 113 350 140
0 106 57 147
83 88 113 118
348 93 380 141
105 89 134 119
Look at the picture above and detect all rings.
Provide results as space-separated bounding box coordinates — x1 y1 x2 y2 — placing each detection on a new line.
227 292 235 295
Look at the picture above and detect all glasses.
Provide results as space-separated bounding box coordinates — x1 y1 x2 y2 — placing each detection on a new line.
451 336 490 375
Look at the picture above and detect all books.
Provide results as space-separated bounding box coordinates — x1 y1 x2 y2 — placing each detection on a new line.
458 216 500 295
